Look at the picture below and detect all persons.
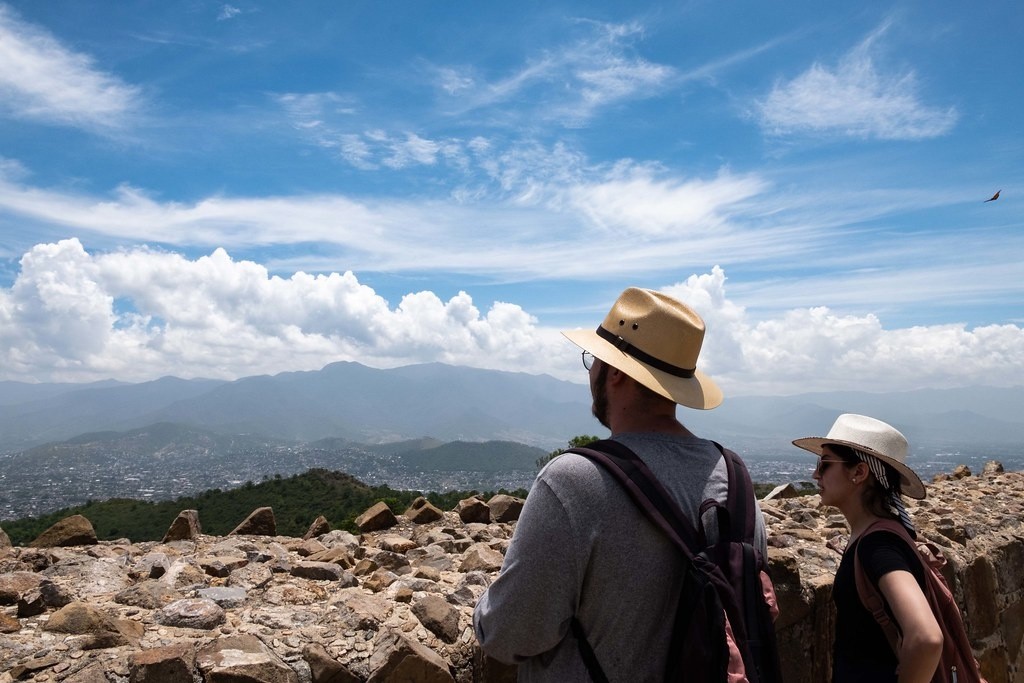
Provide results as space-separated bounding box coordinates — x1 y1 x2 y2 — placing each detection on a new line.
789 410 955 683
470 278 767 682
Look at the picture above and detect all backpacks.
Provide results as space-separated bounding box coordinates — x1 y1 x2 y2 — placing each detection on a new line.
853 519 984 683
564 437 781 683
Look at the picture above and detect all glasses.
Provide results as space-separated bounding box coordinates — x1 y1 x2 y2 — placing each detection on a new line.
582 350 595 370
817 455 850 473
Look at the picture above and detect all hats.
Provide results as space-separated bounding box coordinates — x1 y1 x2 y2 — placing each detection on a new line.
790 413 927 500
560 286 724 409
